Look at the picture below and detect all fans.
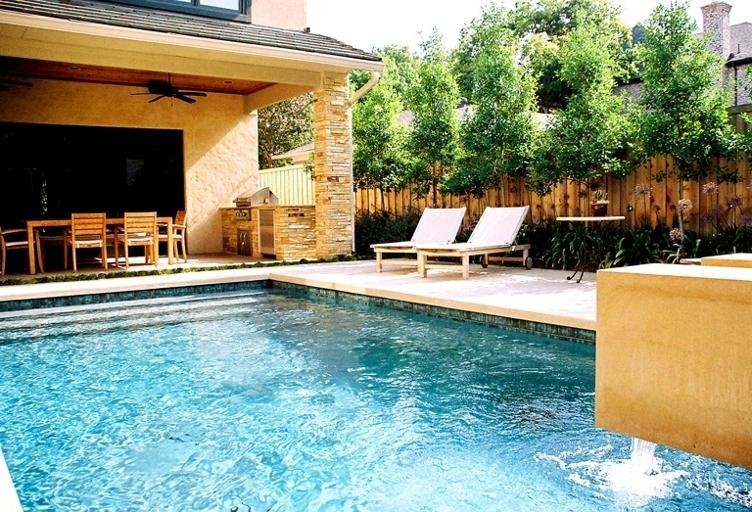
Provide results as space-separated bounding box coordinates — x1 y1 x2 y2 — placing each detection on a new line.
130 73 208 105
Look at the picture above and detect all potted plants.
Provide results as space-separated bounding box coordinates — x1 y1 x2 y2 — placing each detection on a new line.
591 190 610 217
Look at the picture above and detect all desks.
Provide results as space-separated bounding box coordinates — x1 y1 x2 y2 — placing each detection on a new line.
556 216 627 283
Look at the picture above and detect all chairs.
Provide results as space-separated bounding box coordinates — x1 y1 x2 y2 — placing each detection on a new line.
0 208 193 278
370 204 533 280
